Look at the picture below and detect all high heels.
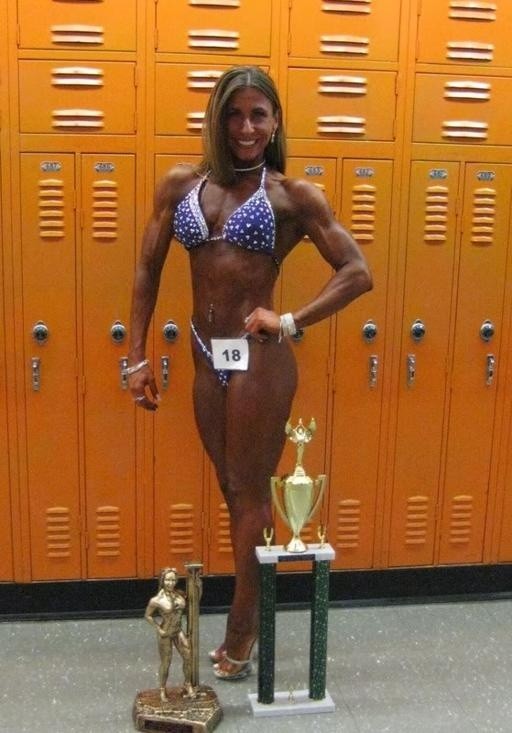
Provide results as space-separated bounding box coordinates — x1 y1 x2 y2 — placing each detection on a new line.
208 614 260 682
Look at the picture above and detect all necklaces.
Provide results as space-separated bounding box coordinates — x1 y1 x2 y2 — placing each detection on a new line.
231 159 266 172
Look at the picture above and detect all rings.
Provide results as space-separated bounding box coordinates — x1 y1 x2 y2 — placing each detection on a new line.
244 317 249 323
136 395 146 402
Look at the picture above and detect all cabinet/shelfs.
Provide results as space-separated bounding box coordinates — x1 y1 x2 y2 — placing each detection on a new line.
208 153 263 579
76 144 141 582
336 154 394 575
277 151 336 582
285 2 400 147
150 148 209 580
7 142 86 583
389 158 464 569
154 1 281 141
410 5 512 152
7 1 145 141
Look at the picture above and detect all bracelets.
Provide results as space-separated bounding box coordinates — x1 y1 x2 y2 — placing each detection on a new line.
121 360 150 377
278 312 298 344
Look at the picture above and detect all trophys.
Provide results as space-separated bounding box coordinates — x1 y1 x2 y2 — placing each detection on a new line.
269 415 328 553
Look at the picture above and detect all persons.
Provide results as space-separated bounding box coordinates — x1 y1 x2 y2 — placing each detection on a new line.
126 66 372 674
145 568 203 702
